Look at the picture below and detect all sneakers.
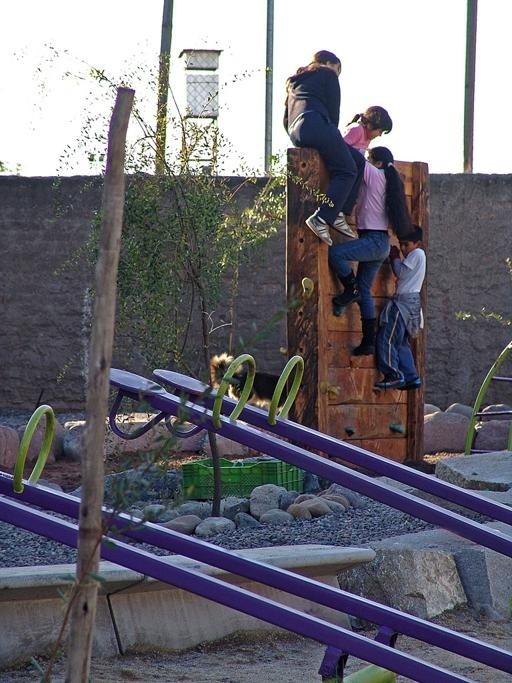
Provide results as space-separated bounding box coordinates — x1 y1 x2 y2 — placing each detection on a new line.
305 209 333 247
374 370 406 387
396 377 422 389
332 213 360 239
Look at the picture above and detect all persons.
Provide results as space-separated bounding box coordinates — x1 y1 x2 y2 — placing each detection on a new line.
343 105 393 157
283 50 366 247
374 224 427 391
328 145 415 356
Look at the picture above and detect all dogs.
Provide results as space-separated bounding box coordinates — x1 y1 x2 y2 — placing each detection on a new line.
209 352 287 415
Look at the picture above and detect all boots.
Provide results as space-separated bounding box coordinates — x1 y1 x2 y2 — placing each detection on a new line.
333 270 361 306
352 318 377 356
